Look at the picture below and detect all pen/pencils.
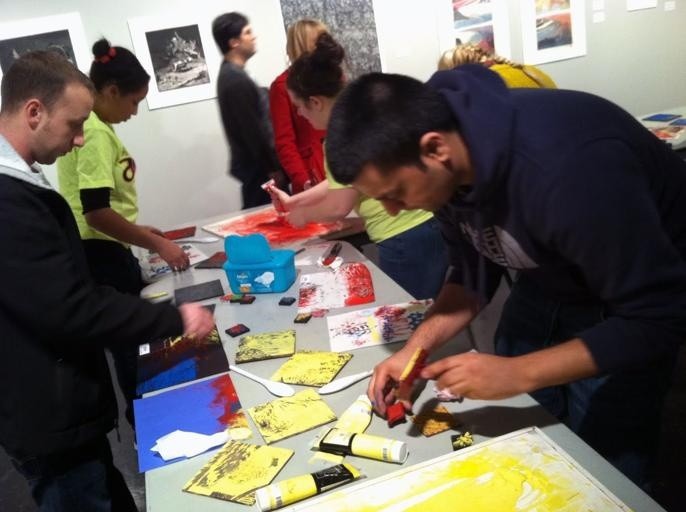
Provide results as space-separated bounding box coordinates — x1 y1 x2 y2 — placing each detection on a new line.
147 291 168 300
295 247 305 255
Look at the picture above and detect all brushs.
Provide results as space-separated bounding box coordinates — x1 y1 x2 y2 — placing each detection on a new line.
399 346 441 389
260 178 284 213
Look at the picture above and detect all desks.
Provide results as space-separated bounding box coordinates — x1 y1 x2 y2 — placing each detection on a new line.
638 107 686 149
129 204 365 283
139 240 667 512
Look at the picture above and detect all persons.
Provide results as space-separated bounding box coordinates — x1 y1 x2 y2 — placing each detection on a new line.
326 73 686 498
440 45 558 88
209 13 290 210
270 30 448 298
52 38 191 456
268 20 347 192
0 47 215 507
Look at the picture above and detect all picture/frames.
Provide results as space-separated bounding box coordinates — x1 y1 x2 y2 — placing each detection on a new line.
272 425 636 512
435 0 511 66
0 11 94 86
520 0 587 66
127 6 223 112
